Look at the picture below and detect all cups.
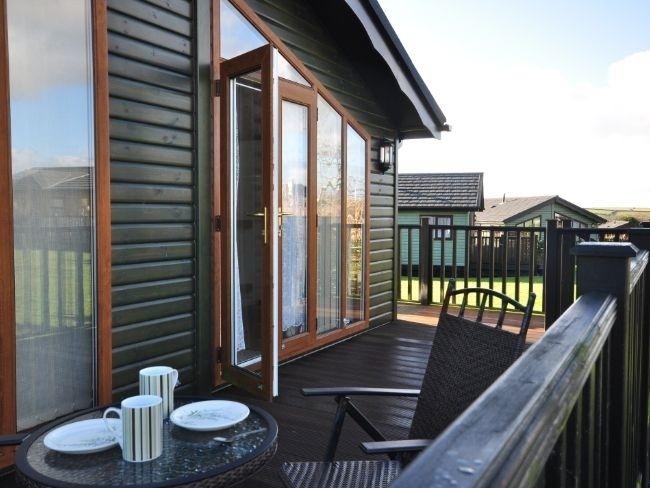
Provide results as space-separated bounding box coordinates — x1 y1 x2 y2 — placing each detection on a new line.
104 395 163 461
139 366 178 419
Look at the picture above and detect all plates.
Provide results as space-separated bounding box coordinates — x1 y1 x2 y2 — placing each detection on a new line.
43 418 121 454
170 400 250 430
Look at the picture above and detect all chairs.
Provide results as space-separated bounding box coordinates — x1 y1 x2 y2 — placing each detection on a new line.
1 428 39 488
278 278 537 487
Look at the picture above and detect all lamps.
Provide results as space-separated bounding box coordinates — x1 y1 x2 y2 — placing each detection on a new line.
378 136 394 174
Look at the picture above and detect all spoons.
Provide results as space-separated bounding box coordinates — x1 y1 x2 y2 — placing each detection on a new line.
213 428 266 442
